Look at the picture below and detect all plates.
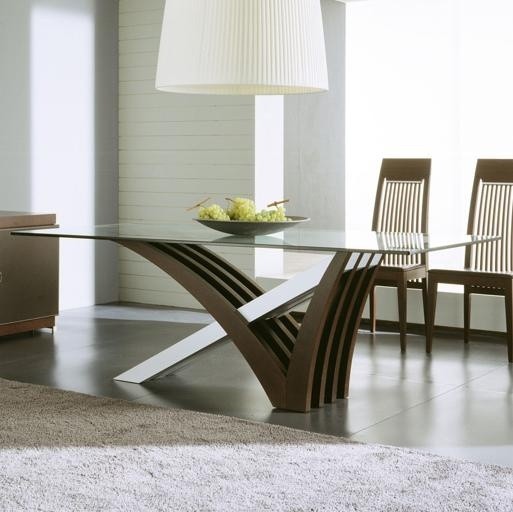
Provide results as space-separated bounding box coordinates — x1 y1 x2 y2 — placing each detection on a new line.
192 215 312 237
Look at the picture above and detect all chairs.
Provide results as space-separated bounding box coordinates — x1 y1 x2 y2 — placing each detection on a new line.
350 158 434 351
421 158 512 362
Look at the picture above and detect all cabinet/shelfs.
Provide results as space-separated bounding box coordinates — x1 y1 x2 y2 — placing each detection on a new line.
0 210 59 338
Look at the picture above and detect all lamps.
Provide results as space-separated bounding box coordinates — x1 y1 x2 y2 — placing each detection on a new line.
155 1 329 98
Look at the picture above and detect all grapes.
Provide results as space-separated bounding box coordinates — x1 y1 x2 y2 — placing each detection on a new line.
197 197 292 222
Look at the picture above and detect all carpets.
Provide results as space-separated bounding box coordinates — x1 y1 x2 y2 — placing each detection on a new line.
1 379 511 512
